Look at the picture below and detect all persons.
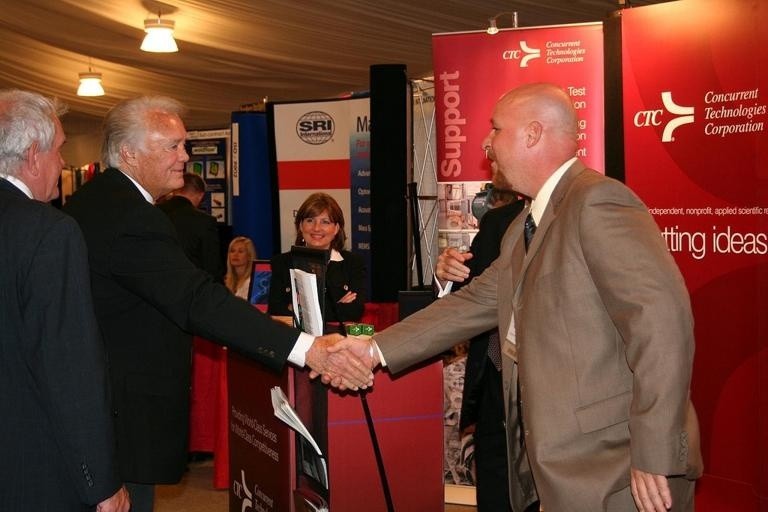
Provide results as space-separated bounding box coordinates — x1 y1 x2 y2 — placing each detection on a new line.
61 95 375 512
434 199 540 512
154 172 225 282
0 89 133 512
309 82 704 512
268 192 368 328
213 236 256 301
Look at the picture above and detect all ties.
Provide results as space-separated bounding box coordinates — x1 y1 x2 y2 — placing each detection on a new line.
523 212 537 256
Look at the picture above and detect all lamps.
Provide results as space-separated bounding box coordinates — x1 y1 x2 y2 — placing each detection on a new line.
140 7 179 53
487 11 518 35
77 68 106 97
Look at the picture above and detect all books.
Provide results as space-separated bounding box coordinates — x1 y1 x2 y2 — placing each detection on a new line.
269 386 329 490
288 268 324 336
